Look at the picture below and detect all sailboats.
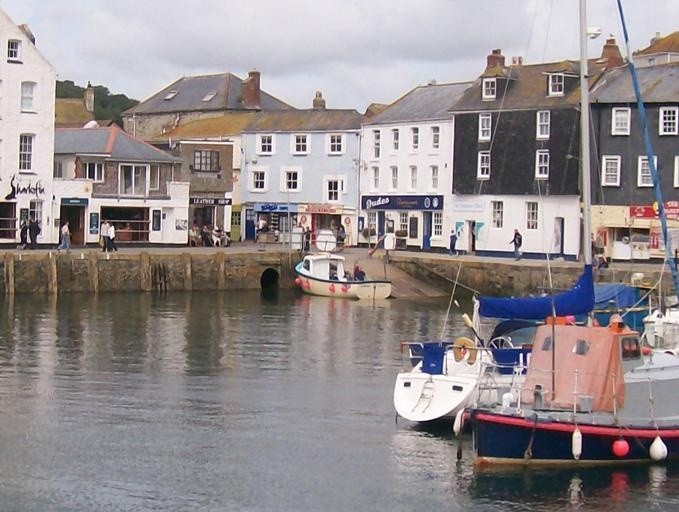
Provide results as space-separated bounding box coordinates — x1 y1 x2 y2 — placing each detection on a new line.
387 0 678 422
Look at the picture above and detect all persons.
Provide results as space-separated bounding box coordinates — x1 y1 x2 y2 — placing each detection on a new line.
188 224 232 248
509 229 522 261
354 266 365 281
57 221 73 254
304 227 310 252
29 218 40 249
598 257 608 268
449 230 458 257
107 222 118 253
338 224 346 246
100 218 113 253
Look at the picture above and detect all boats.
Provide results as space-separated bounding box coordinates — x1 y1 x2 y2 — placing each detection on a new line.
292 249 394 305
454 403 679 482
403 279 668 384
